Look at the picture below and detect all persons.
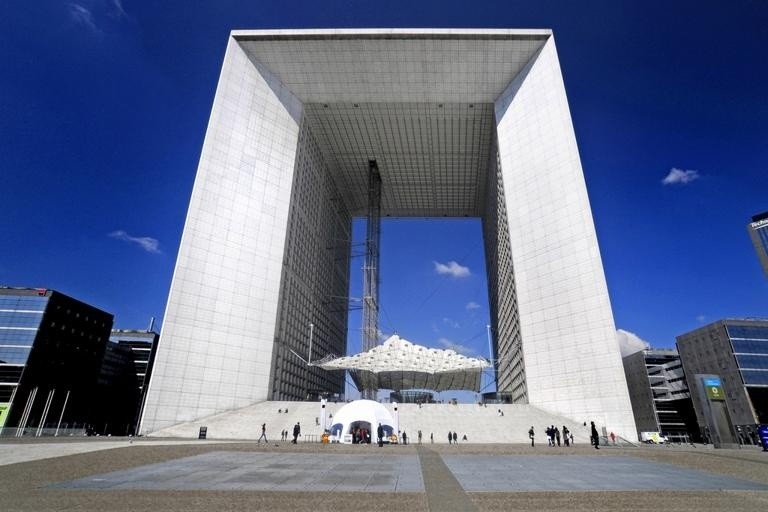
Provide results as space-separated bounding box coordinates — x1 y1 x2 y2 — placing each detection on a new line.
591 420 600 449
528 426 535 447
543 424 574 447
453 432 458 445
281 429 285 441
257 423 269 443
290 422 301 444
352 421 425 447
430 432 434 444
284 430 288 441
447 430 453 444
315 416 320 425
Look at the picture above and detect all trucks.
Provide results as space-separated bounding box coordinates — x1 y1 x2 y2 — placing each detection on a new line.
641 430 669 446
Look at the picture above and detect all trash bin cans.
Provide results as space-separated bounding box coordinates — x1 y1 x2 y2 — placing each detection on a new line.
199 427 207 439
